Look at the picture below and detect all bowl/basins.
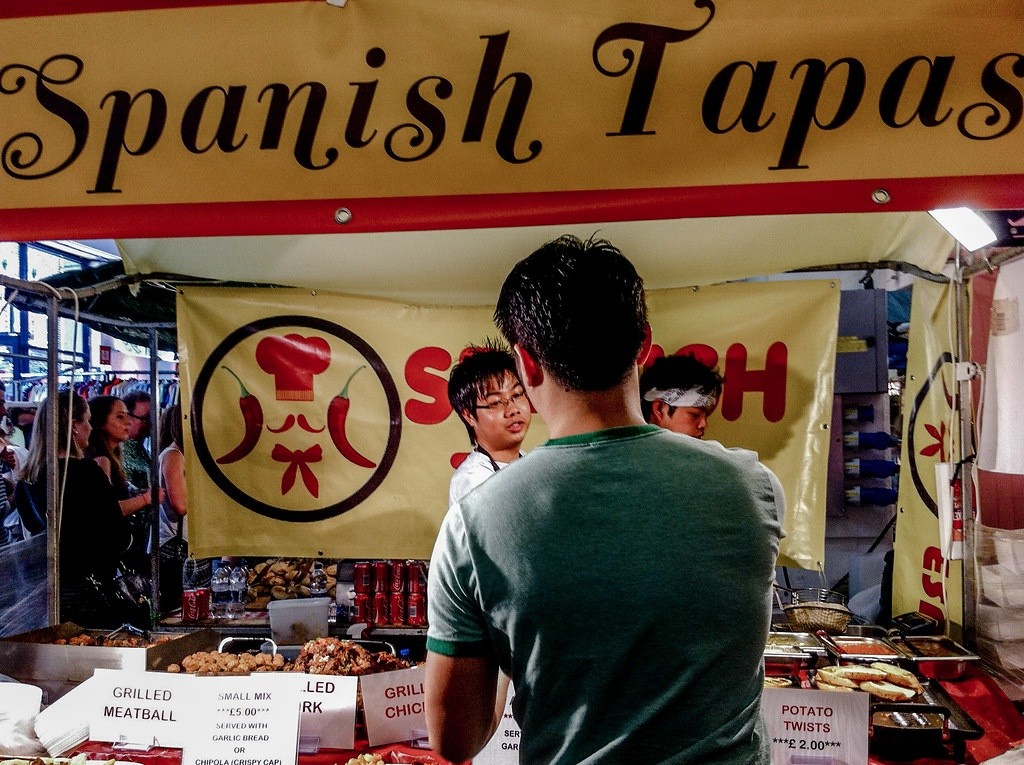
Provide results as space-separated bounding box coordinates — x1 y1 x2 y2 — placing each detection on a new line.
764 631 981 680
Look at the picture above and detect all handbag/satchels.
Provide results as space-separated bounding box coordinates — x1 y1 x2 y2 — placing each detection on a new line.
82 570 152 631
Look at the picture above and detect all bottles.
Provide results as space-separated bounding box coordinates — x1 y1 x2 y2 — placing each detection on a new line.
399 648 417 667
223 560 231 575
310 563 328 599
229 564 246 619
210 562 229 619
239 560 250 578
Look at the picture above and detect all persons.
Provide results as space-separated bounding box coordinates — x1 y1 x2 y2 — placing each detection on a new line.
447 351 531 507
424 235 787 765
0 380 189 627
638 354 720 440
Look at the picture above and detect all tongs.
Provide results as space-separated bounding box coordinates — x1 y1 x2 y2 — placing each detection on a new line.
817 629 846 654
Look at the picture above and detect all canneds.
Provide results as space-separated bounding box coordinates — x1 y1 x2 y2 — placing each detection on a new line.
180 587 209 622
353 561 427 627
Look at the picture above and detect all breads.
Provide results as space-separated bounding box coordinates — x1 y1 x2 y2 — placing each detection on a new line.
812 662 923 703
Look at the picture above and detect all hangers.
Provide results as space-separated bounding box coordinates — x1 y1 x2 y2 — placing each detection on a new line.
28 372 182 387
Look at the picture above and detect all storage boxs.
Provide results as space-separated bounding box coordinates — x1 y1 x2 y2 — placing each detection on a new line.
0 622 191 679
978 530 1024 671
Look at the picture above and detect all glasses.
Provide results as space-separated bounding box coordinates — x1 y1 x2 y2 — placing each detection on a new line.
127 413 150 424
473 384 525 409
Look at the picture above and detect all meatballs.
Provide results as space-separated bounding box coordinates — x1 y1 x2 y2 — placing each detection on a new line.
167 650 285 672
